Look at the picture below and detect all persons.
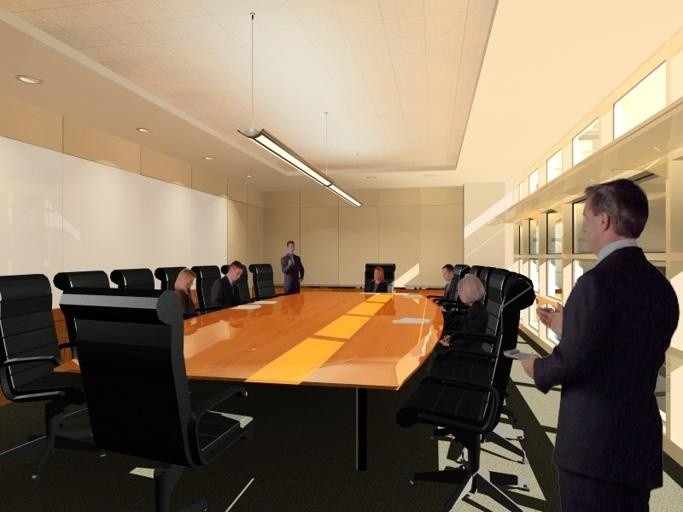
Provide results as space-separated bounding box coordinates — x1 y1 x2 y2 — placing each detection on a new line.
521 179 678 511
368 266 386 292
438 275 485 354
173 268 196 319
280 241 303 293
210 261 244 310
436 266 458 305
